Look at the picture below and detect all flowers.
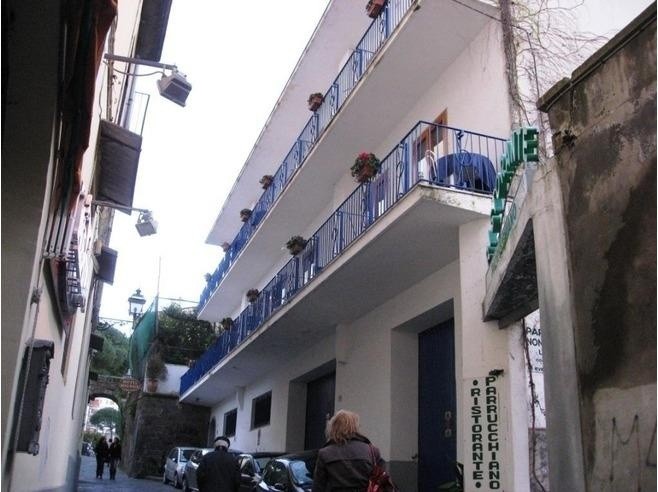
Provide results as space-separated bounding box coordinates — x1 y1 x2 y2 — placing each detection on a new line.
349 149 381 177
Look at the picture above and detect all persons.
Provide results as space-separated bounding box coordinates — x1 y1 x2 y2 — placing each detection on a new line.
311 409 385 492
92 436 108 479
109 437 121 480
196 435 241 492
106 438 113 466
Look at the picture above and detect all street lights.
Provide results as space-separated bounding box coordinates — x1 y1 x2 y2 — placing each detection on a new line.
128 289 147 330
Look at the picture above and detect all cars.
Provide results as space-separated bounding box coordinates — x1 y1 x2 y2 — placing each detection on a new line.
182 448 318 492
163 446 199 489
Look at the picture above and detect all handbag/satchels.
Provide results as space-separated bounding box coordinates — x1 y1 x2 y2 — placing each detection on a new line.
366 443 395 492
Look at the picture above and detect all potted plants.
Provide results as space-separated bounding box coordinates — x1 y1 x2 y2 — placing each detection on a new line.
219 317 234 330
286 235 308 255
246 289 259 302
240 207 251 221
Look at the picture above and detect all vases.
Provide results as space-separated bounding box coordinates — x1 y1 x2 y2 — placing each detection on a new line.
355 165 374 182
